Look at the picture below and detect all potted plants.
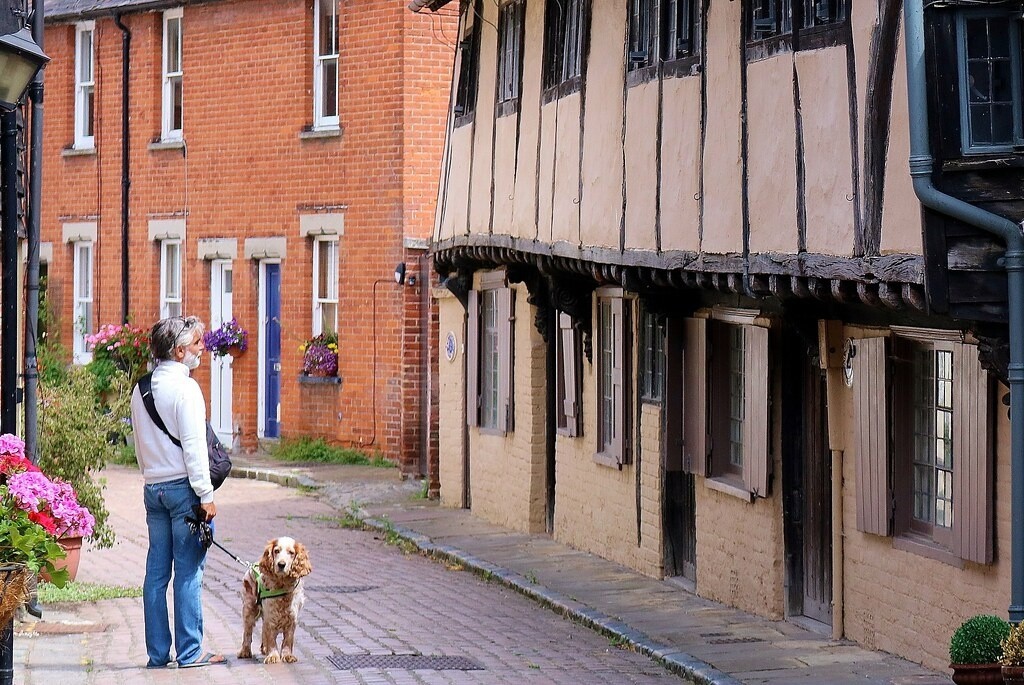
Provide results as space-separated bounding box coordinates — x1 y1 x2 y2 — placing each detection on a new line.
996 620 1024 684
947 614 1011 685
7 474 96 588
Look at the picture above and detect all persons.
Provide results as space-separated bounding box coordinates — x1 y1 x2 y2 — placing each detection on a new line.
131 316 232 666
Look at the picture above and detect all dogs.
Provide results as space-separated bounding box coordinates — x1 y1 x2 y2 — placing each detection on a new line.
236 536 312 665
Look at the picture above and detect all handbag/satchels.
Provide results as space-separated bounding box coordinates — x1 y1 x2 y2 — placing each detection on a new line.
137 371 232 492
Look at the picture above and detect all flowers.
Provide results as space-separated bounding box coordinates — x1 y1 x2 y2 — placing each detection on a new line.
203 316 248 361
299 326 338 373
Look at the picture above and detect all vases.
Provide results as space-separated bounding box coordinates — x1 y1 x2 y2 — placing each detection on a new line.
228 344 245 358
312 370 337 377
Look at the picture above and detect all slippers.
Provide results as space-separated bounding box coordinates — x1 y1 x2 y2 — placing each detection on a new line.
147 648 227 669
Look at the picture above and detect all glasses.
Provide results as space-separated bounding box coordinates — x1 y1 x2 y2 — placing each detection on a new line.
172 316 195 348
